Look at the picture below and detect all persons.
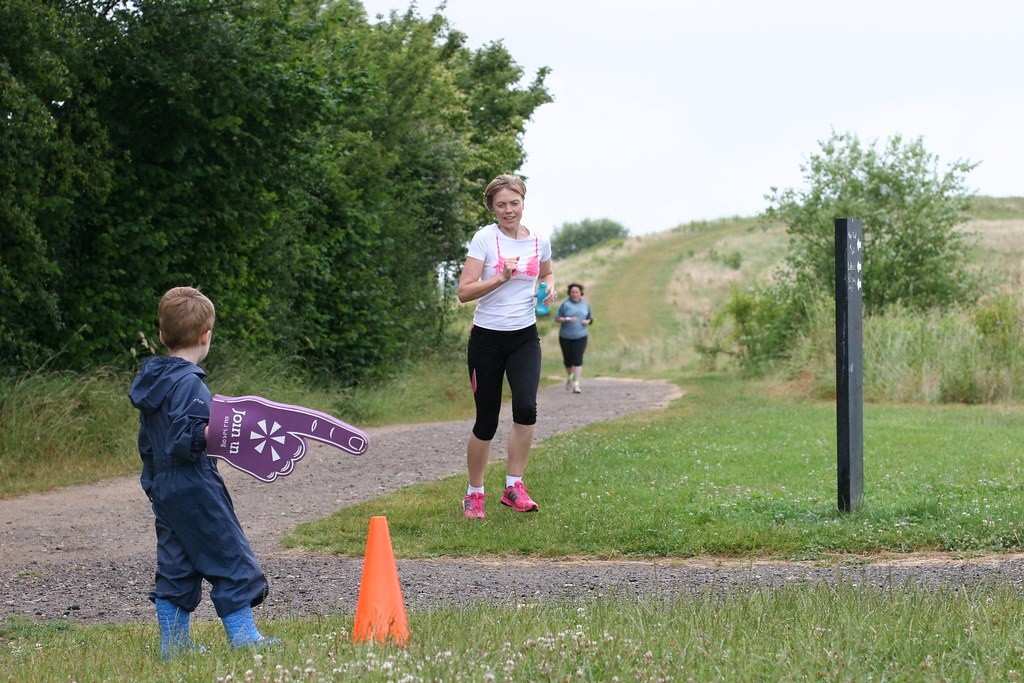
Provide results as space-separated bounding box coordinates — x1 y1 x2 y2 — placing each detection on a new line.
555 283 593 394
127 285 280 653
456 174 559 521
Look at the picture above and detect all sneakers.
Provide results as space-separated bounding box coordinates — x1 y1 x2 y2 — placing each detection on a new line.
461 491 486 520
566 372 581 393
501 480 539 512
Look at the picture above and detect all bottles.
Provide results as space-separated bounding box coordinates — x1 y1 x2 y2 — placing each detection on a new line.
536 282 550 314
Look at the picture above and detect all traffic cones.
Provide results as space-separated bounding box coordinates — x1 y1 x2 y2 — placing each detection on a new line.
355 514 410 646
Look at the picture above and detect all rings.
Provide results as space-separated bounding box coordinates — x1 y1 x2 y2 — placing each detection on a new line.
554 294 558 298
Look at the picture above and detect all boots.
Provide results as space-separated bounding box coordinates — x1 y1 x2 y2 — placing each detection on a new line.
154 597 206 661
222 603 282 652
